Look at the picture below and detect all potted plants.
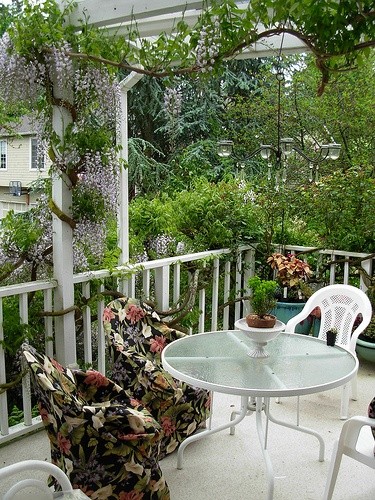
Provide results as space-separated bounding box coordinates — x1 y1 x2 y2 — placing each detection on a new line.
260 253 313 333
353 315 375 363
245 275 280 327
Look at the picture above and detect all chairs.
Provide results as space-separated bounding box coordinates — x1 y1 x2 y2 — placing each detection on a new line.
0 284 375 500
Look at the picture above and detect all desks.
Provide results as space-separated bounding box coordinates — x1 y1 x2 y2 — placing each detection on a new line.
162 330 360 500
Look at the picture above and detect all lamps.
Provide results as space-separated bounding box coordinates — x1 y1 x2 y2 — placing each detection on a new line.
217 54 341 194
